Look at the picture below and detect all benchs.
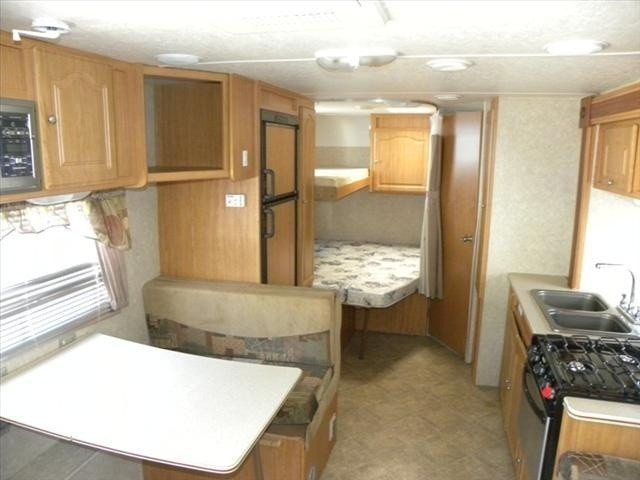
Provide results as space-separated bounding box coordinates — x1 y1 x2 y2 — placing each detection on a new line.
143 273 340 480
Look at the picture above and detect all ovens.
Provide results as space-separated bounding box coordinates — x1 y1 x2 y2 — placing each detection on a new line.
507 359 556 475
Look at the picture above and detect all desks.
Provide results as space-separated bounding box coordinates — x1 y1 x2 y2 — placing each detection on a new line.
0 330 304 480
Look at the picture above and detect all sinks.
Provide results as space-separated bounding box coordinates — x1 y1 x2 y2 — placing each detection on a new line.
532 289 613 311
547 310 638 335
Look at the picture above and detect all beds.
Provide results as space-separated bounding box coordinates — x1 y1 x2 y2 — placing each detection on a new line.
314 237 428 361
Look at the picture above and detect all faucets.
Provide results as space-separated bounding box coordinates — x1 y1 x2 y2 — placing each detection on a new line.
595 262 636 311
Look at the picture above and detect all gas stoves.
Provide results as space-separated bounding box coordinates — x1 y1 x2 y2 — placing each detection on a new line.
527 333 640 417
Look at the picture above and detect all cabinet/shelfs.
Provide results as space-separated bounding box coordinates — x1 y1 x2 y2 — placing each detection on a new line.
138 67 259 184
366 110 432 193
581 82 640 200
298 101 317 286
498 280 533 480
1 32 146 203
